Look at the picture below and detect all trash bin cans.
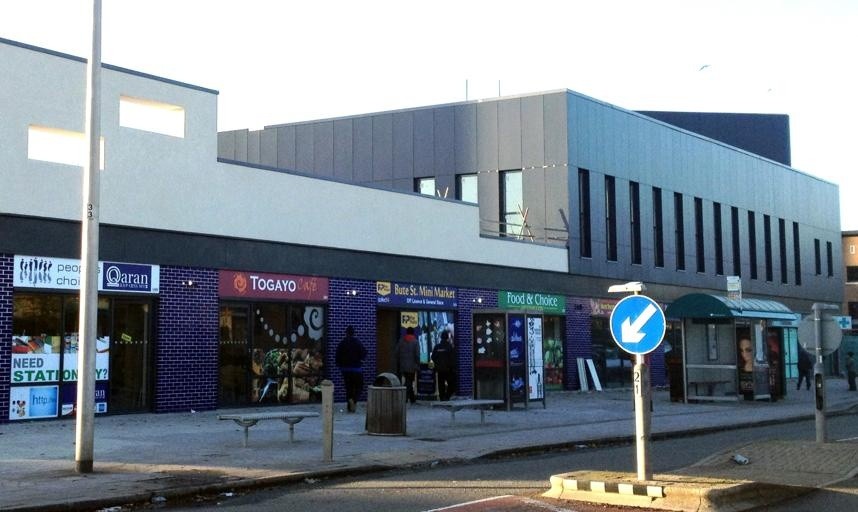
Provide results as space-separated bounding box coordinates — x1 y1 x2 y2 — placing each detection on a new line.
365 372 408 437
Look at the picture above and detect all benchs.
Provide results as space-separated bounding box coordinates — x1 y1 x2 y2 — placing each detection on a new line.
218 410 319 447
430 398 505 425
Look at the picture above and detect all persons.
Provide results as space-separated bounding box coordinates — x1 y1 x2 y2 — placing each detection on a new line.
334 324 368 413
430 330 458 401
845 352 857 390
392 327 421 403
738 334 753 392
796 348 812 390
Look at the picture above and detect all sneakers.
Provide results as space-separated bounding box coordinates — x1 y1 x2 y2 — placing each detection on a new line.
349 398 355 412
